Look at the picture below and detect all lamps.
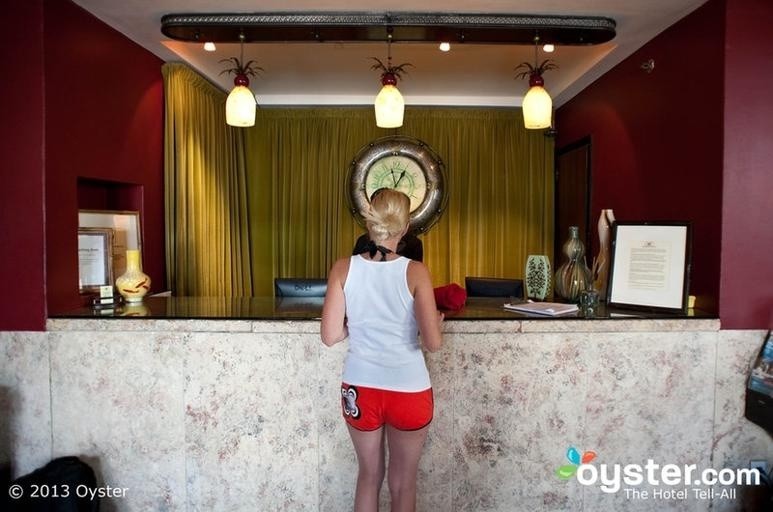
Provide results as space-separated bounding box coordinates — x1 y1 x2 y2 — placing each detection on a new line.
215 35 262 130
366 38 415 131
512 38 561 132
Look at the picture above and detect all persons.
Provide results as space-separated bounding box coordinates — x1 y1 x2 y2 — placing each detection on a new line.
354 190 425 262
320 190 444 512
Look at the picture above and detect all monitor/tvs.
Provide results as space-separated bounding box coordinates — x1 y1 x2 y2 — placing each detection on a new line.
274 277 328 297
465 277 524 297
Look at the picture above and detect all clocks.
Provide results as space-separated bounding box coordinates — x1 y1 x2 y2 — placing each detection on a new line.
340 134 451 240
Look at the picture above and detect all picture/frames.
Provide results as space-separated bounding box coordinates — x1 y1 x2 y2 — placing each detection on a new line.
76 226 115 293
603 218 694 317
74 208 145 290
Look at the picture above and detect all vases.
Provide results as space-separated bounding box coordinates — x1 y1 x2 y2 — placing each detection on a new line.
593 209 613 301
113 250 152 304
525 253 553 301
554 225 595 300
